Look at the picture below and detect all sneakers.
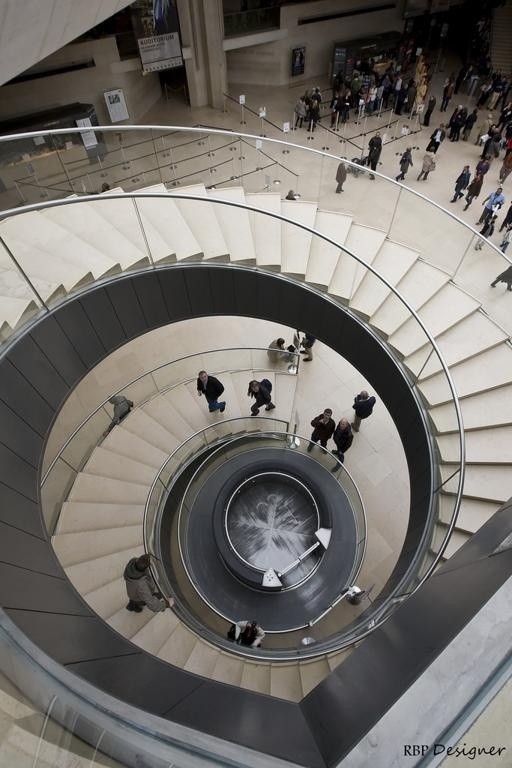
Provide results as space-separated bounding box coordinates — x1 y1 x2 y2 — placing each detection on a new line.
300 351 312 361
308 445 338 472
220 402 275 416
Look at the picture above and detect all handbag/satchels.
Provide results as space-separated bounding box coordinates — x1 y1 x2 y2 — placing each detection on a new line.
495 150 499 157
261 379 272 393
302 340 310 348
428 163 435 171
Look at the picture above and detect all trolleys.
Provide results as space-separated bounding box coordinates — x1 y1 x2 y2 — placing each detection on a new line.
283 329 300 362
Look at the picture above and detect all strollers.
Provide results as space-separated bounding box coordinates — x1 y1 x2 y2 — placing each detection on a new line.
346 155 369 177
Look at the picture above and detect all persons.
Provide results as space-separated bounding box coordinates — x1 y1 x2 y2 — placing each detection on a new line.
286 191 296 200
103 394 134 437
124 554 174 614
279 345 296 373
331 417 354 472
247 380 275 416
293 17 511 292
101 182 111 191
234 620 265 650
300 332 316 362
196 371 226 412
307 409 335 455
266 338 285 366
350 390 376 432
